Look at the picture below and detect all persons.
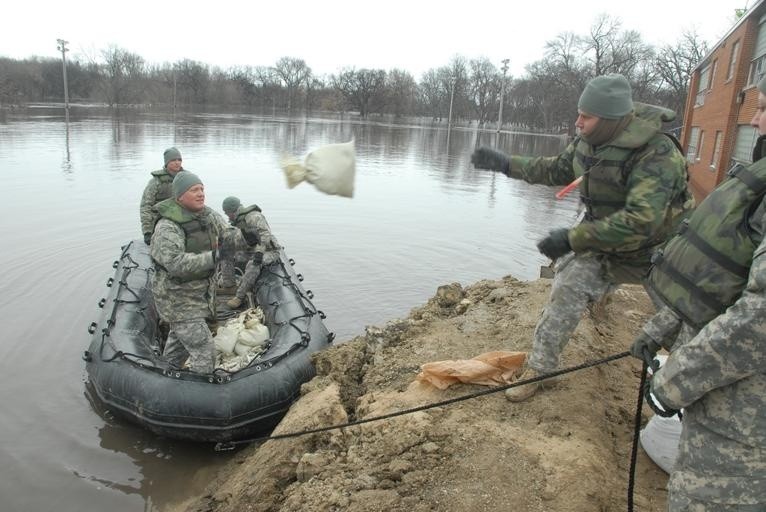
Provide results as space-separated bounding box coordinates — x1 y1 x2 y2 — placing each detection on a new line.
219 194 282 309
468 71 697 404
629 72 766 510
138 146 184 247
143 169 247 377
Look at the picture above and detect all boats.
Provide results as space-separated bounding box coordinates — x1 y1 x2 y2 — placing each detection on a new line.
82 234 336 452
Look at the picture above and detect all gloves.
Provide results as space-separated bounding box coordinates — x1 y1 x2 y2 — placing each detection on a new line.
217 229 238 262
629 330 661 359
470 146 509 176
535 228 570 262
143 232 153 246
253 252 262 266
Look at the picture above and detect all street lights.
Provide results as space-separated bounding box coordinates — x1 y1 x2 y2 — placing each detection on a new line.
448 75 456 130
497 58 510 130
57 39 71 109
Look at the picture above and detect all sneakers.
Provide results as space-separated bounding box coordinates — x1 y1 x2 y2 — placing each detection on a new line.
505 368 559 403
215 287 236 296
227 297 242 309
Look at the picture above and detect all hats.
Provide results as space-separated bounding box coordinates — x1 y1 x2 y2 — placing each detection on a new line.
172 171 205 203
163 148 183 167
222 196 241 213
577 72 635 121
